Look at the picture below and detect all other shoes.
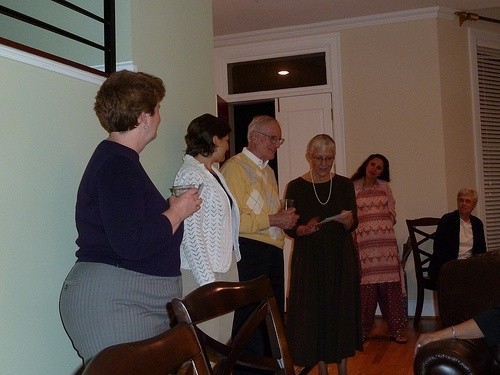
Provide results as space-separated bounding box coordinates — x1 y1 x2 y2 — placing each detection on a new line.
396 336 408 344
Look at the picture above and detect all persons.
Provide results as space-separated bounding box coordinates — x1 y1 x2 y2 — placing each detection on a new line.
284 134 364 375
172 113 241 355
429 189 485 277
351 154 408 343
219 115 299 359
415 310 500 353
60 69 203 366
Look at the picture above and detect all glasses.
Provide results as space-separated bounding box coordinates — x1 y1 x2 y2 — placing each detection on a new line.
307 154 335 161
256 131 285 145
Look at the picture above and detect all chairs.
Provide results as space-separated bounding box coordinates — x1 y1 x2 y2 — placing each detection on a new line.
82 321 206 375
405 217 439 327
171 272 296 375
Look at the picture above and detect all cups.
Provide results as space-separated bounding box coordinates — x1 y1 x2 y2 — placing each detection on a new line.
172 184 194 216
279 199 295 210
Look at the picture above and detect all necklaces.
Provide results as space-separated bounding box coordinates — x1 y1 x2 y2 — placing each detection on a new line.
310 168 332 205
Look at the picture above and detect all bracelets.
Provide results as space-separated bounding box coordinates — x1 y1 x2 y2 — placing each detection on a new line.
451 325 455 342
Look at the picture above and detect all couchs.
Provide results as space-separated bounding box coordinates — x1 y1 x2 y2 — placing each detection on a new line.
414 250 500 375
